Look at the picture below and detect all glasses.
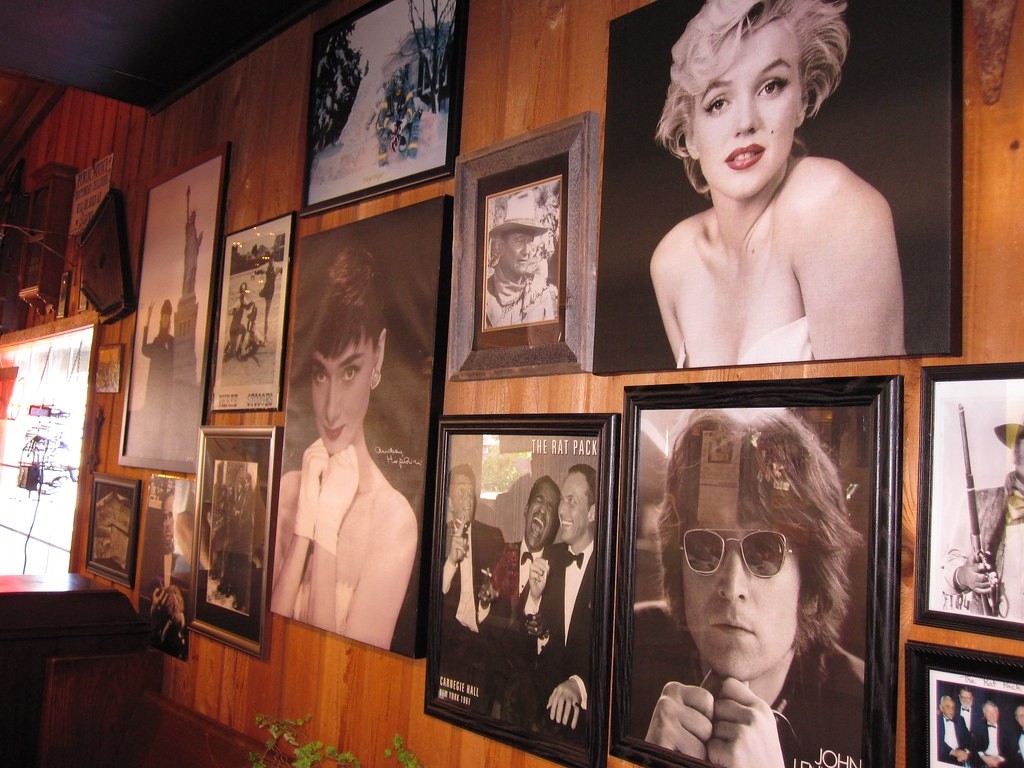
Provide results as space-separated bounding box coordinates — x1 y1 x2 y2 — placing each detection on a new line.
674 528 801 579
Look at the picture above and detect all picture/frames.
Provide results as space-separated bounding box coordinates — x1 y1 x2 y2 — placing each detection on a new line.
609 372 903 768
193 425 286 660
118 139 232 473
210 211 298 412
423 411 620 767
913 362 1024 641
299 1 470 218
904 639 1024 768
444 110 599 380
85 471 141 590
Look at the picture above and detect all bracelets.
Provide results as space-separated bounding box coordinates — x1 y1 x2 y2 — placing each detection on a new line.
956 567 966 589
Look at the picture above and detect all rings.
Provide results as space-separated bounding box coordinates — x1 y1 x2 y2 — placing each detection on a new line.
575 703 580 707
535 627 538 632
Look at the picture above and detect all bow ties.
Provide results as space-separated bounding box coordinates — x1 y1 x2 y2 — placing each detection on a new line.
561 551 584 569
520 552 533 565
162 544 175 555
453 517 470 540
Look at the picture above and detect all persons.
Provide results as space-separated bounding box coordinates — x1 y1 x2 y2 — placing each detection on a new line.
650 0 906 369
485 190 558 328
627 407 866 768
141 491 194 646
365 67 423 168
182 185 203 295
942 407 1024 622
443 463 596 739
937 687 1024 768
212 462 256 610
270 240 417 649
222 256 277 363
141 299 175 459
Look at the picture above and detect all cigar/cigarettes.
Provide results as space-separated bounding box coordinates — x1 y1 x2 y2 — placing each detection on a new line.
481 569 493 577
455 517 467 528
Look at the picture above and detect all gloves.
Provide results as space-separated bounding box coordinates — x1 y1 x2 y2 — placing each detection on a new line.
313 445 360 555
293 437 329 543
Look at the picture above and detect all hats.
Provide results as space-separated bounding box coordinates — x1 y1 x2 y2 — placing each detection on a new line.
994 421 1024 447
489 190 550 238
160 299 172 315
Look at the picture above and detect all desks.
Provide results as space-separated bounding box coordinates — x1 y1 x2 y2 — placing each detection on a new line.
0 583 153 767
